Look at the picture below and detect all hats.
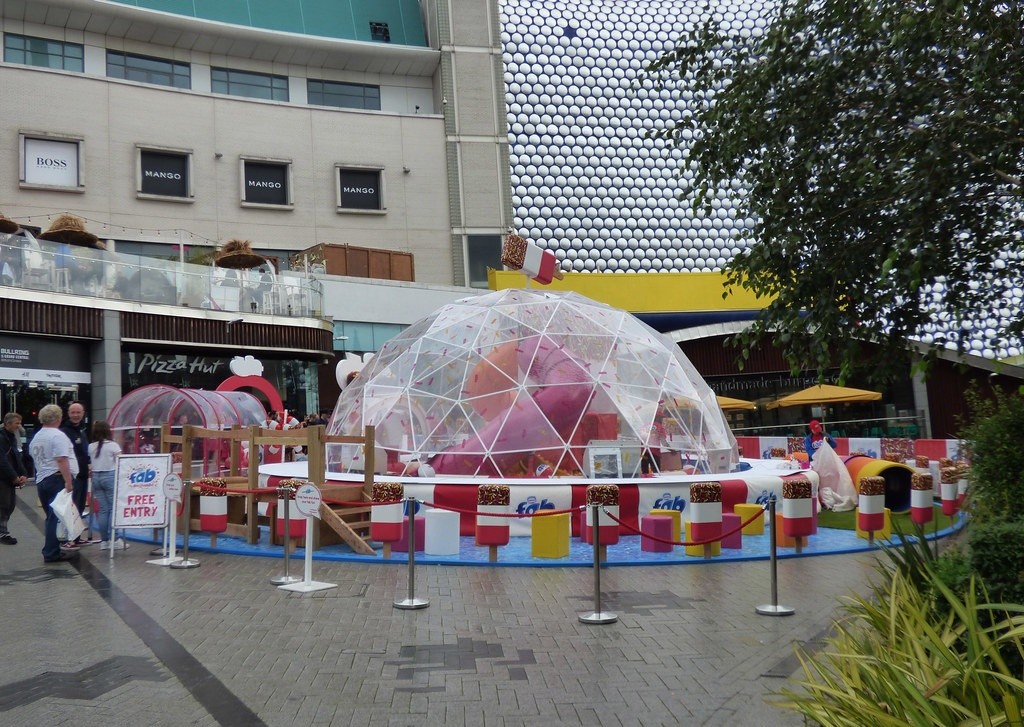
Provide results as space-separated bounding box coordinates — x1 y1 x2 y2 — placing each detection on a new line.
809 420 822 432
657 411 667 417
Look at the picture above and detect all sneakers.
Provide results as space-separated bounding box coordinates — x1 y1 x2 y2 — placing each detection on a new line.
100 540 110 550
109 538 130 550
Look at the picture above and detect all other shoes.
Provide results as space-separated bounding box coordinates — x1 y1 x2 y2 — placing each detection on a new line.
76 538 91 546
48 550 78 562
61 543 81 549
0 530 17 545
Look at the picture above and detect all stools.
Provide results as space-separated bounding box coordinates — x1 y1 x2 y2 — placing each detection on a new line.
56 268 71 293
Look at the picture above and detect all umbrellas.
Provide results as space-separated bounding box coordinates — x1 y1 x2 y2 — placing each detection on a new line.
216 241 264 268
0 214 18 234
37 214 97 246
661 393 755 434
91 241 119 260
767 383 882 434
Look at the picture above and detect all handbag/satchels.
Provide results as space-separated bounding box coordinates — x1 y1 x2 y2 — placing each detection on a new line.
49 488 86 542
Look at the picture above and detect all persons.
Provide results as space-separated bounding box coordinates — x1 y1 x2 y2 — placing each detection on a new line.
55 240 79 283
804 420 836 461
253 268 272 304
87 420 130 550
265 409 333 455
59 400 92 550
0 412 28 545
124 409 256 459
28 404 81 562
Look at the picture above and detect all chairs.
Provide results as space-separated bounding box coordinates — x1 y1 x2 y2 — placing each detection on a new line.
21 248 53 290
263 291 308 316
